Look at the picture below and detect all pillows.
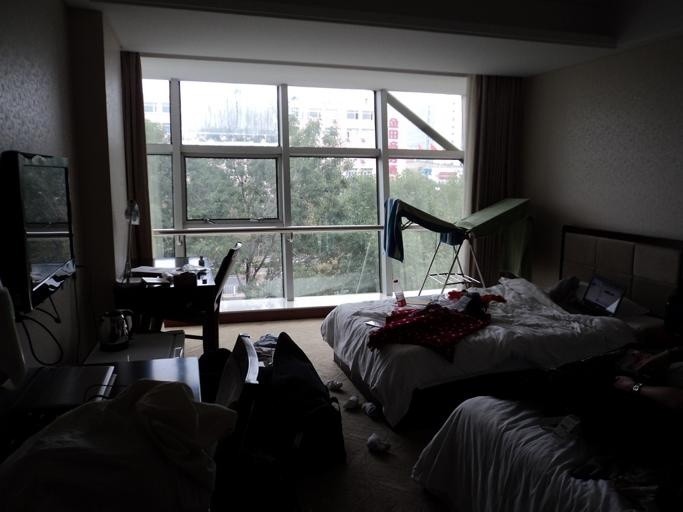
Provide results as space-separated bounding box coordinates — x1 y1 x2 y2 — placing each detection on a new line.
545 271 652 319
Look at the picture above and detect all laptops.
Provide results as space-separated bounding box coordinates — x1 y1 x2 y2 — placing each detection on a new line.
562 274 626 317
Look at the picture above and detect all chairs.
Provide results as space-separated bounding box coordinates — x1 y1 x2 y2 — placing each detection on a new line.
152 241 242 349
213 335 260 410
411 339 683 509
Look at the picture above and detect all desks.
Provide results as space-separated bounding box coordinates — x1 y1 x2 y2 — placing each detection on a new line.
119 257 217 353
0 356 202 461
80 327 186 364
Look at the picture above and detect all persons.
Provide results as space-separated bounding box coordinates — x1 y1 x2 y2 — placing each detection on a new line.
612 341 683 410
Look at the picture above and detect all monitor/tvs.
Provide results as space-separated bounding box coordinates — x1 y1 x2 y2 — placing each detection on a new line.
0 151 76 313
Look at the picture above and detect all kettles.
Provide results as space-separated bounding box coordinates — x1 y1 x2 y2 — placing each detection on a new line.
97 307 136 353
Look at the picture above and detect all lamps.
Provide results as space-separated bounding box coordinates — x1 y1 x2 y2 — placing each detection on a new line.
123 198 140 284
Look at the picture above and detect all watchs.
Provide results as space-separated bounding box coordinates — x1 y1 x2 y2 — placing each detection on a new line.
631 382 645 394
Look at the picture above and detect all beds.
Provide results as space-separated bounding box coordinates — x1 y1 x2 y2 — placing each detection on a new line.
317 226 682 432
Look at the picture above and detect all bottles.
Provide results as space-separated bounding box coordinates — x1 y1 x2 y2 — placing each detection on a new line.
392 279 407 309
198 255 204 266
